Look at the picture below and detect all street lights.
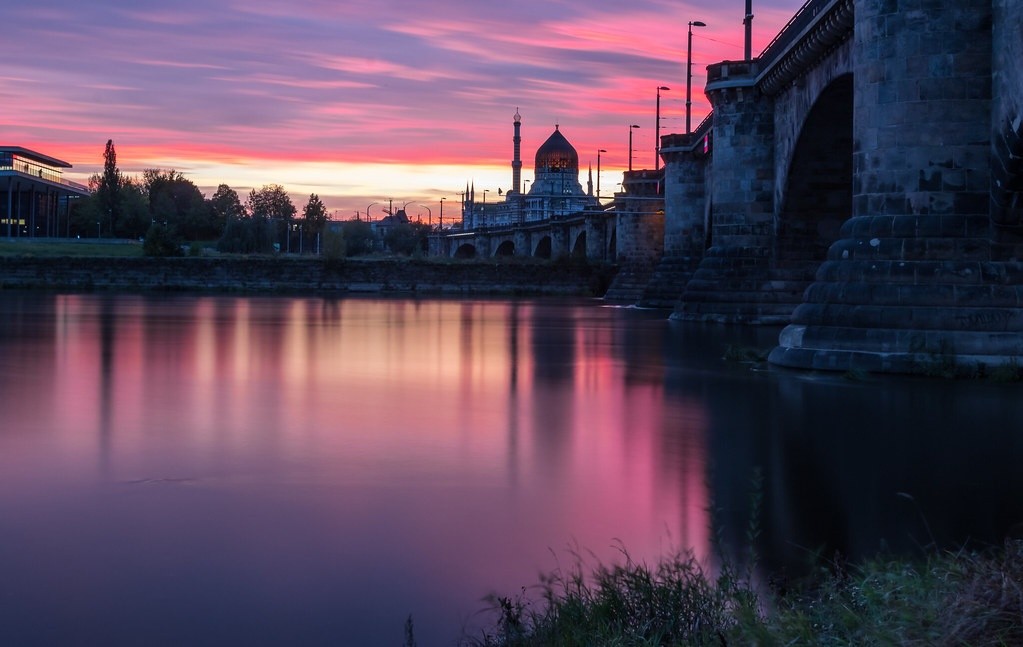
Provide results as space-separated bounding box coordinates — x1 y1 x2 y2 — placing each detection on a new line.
97 222 101 240
597 148 606 205
299 223 303 254
483 189 489 230
562 157 568 214
524 179 530 195
440 197 446 232
287 220 291 255
629 123 639 173
685 21 704 134
656 85 671 171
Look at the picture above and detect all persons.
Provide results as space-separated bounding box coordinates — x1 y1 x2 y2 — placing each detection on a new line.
39 169 42 178
